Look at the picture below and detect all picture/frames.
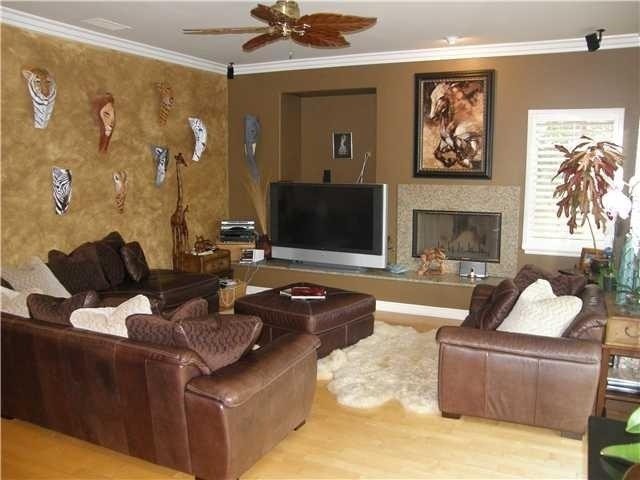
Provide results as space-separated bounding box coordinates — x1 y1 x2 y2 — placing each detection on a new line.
412 67 497 180
331 131 353 160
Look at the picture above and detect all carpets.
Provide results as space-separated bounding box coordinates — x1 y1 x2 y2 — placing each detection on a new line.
316 321 439 415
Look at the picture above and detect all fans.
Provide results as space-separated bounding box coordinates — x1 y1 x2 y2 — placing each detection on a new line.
179 2 377 52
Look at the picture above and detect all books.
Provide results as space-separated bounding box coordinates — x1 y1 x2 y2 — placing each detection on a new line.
290 285 327 300
280 287 292 297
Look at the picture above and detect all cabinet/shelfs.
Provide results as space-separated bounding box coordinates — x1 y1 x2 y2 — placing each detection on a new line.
597 315 640 417
233 258 503 321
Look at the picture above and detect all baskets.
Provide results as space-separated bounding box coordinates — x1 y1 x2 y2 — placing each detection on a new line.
218 280 247 308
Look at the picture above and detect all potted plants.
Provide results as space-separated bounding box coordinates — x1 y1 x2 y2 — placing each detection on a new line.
551 136 627 275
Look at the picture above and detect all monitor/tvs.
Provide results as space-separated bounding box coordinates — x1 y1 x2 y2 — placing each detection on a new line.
270 182 388 270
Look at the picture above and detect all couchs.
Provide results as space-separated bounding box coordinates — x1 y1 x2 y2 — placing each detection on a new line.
437 282 608 438
1 230 322 479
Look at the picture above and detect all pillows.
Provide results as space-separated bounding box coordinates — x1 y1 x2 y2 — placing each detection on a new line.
1 240 261 374
471 265 588 336
